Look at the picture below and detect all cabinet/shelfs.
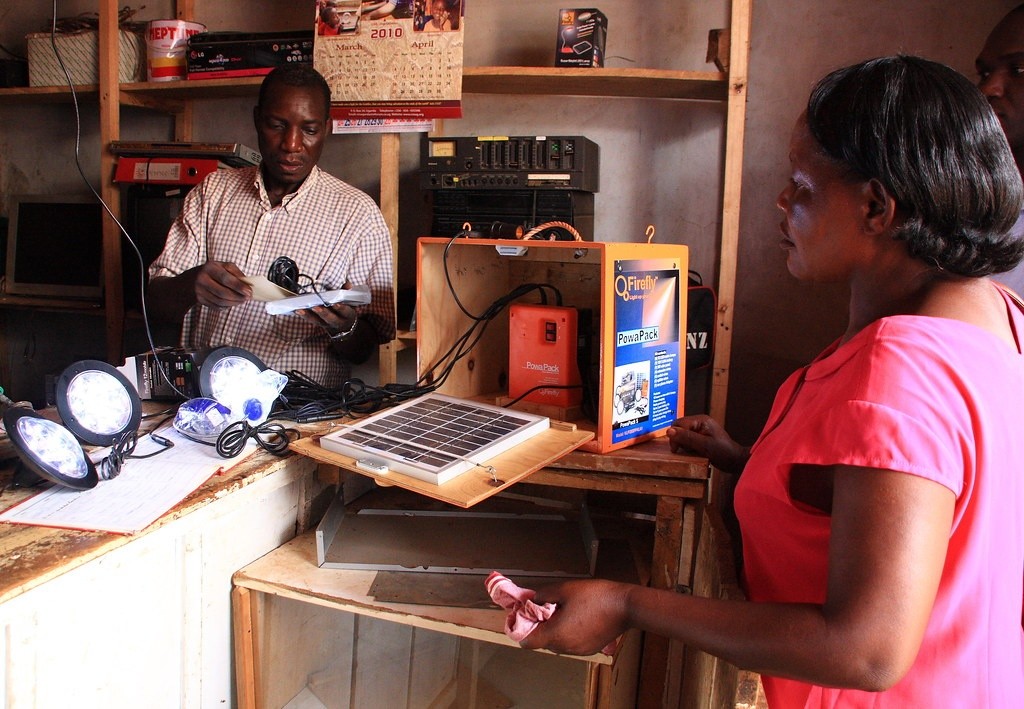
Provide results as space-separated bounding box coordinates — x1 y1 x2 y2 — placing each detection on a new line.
296 404 718 708
0 0 752 460
230 490 653 708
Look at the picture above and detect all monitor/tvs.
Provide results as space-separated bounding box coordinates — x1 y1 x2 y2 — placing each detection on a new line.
128 183 196 306
6 194 103 297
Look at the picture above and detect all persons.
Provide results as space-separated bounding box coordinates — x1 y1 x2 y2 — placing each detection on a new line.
142 60 398 403
508 55 1022 709
974 0 1023 301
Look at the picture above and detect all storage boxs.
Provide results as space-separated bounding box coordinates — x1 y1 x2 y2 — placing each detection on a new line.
136 346 217 400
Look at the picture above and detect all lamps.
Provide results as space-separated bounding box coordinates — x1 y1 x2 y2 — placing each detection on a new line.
55 360 142 446
0 408 100 497
199 347 267 400
